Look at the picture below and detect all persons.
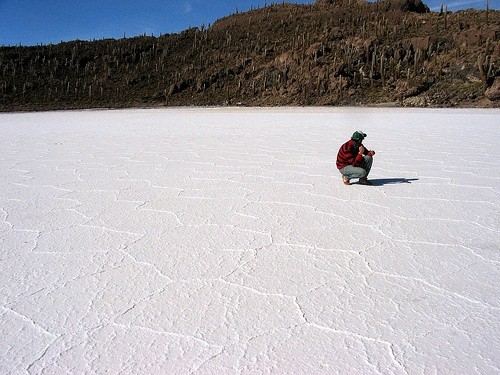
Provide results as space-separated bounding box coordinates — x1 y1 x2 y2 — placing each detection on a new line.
336 131 375 185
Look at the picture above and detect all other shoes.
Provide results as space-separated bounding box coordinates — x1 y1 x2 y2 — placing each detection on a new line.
359 178 372 185
342 174 350 185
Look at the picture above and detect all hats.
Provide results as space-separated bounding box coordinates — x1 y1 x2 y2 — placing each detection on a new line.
353 131 366 140
